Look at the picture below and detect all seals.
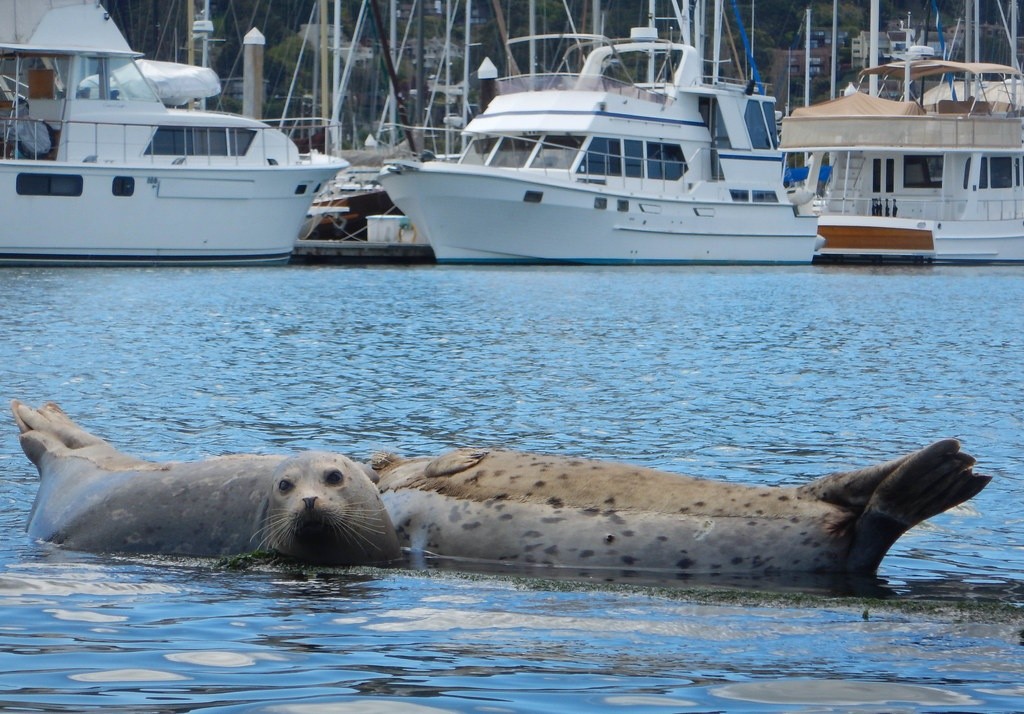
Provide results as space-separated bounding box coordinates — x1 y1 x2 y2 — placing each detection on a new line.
12 400 994 575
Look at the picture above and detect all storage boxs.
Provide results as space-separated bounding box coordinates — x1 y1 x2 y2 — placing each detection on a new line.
26 69 55 99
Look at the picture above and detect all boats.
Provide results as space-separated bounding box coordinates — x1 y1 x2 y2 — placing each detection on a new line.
374 1 825 267
776 0 1024 266
0 0 463 269
